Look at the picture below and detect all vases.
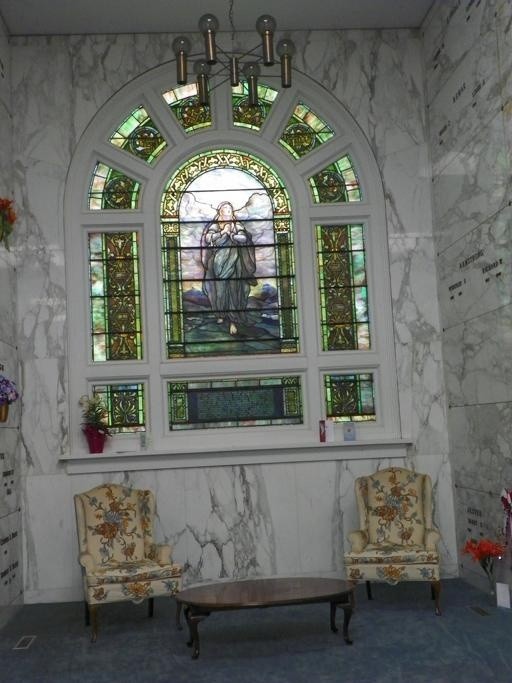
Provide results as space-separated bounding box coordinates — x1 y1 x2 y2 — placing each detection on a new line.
83 428 109 453
488 584 496 607
0 402 8 422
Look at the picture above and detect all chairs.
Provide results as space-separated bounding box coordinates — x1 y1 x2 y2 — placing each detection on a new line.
343 466 441 615
74 483 183 643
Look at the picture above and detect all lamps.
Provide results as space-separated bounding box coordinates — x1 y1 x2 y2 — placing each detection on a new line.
172 0 296 107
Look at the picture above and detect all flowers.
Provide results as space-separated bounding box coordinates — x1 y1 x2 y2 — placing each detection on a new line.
0 375 18 404
0 198 17 252
78 387 113 437
459 532 508 588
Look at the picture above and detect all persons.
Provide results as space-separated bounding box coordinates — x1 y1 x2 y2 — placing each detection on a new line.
200 201 258 335
